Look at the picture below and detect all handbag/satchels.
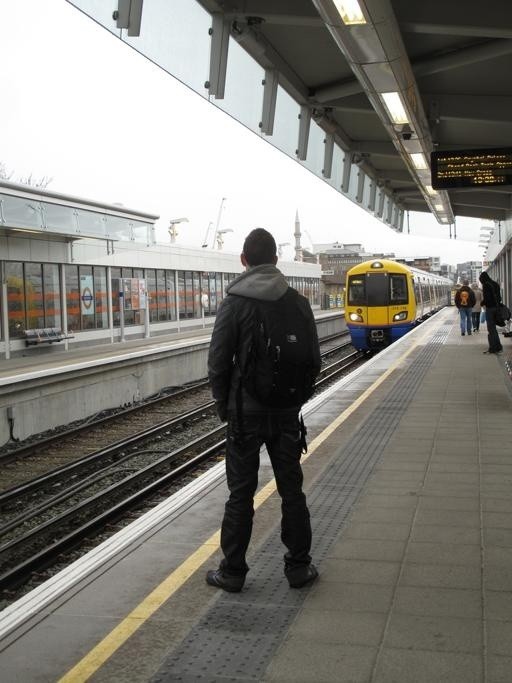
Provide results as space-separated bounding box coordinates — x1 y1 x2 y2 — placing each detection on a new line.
496 304 511 327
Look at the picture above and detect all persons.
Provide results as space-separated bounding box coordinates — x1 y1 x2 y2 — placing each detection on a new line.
455 280 476 335
471 284 483 332
479 272 502 353
207 227 321 590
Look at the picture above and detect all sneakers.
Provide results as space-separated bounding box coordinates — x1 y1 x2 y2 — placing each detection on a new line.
461 327 479 336
206 570 243 593
286 564 319 588
483 349 503 355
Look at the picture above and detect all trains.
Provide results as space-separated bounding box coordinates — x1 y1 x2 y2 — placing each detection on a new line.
342 259 456 352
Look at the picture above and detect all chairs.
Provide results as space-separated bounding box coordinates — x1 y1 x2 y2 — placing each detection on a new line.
21 328 74 346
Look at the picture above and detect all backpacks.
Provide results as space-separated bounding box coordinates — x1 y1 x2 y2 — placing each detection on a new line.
245 286 315 410
459 289 470 306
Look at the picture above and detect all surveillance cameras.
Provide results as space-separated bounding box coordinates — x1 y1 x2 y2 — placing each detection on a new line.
401 124 414 141
